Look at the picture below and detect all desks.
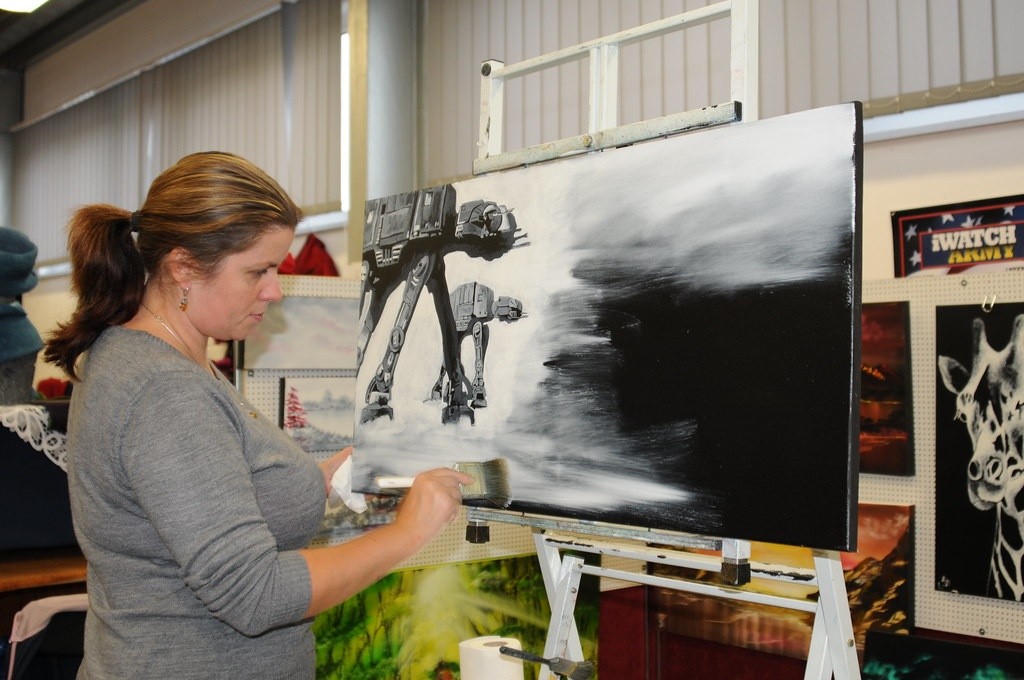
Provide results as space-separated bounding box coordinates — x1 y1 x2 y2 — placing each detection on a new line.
0 558 86 591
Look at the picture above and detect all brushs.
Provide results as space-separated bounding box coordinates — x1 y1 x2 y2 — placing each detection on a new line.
374 456 515 509
499 645 598 680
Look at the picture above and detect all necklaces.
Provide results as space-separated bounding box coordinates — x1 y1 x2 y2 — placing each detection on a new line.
139 301 259 419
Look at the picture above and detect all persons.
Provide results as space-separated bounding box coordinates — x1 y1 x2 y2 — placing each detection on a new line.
43 151 474 680
0 225 80 558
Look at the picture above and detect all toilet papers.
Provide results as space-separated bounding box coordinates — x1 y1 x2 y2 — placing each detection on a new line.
458 635 524 680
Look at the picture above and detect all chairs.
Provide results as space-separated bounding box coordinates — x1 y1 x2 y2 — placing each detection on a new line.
6 594 88 680
